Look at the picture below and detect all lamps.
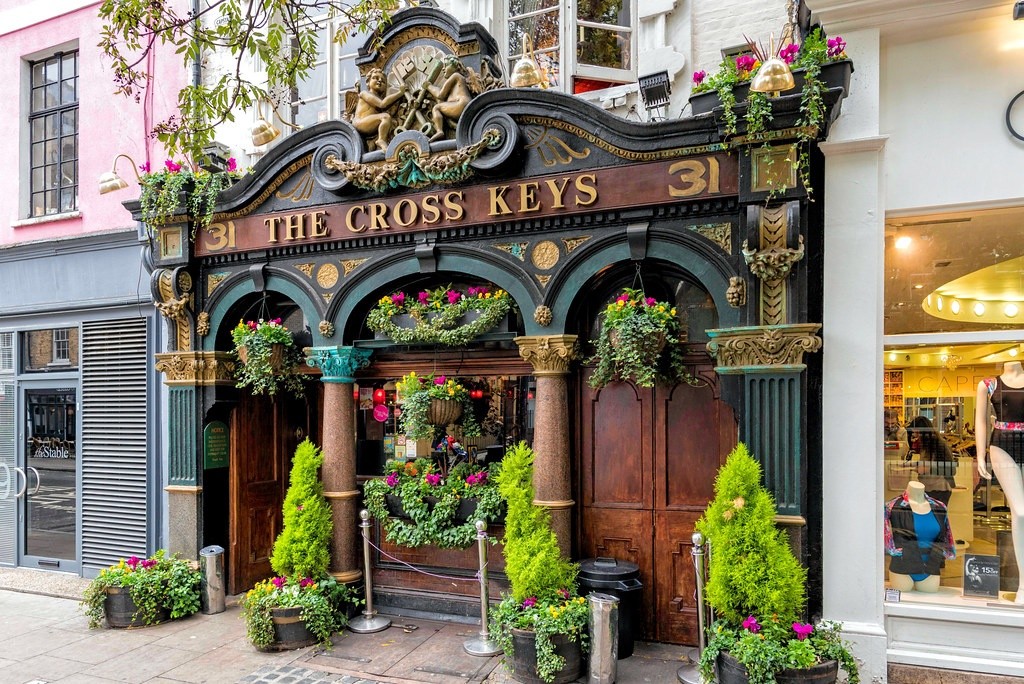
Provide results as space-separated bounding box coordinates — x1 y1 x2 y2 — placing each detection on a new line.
250 95 303 147
750 32 796 97
637 69 671 122
510 32 548 89
1013 1 1024 20
98 154 161 196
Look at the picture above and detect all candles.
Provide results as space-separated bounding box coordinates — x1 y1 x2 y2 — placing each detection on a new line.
580 19 584 42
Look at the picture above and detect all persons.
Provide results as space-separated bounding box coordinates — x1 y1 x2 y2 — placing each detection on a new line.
907 417 957 506
884 481 956 592
884 409 907 441
353 68 408 150
974 362 1024 602
422 53 472 141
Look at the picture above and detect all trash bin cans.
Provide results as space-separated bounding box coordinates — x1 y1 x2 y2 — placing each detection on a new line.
576 557 644 660
199 545 226 615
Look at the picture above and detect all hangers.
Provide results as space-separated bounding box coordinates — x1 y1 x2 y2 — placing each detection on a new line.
947 433 990 453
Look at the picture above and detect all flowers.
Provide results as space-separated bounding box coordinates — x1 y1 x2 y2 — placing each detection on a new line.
138 153 258 244
365 283 514 442
236 436 591 684
577 287 709 392
692 26 848 216
694 440 861 684
78 549 202 629
226 318 314 399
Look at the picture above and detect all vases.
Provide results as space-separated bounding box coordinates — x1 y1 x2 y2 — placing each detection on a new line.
98 579 175 629
374 308 508 340
689 58 855 116
138 178 241 193
718 652 840 684
238 343 284 369
414 397 463 426
383 493 508 526
608 327 667 363
265 604 326 652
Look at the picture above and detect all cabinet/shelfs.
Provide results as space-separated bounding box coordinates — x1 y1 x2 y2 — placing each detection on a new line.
884 369 905 425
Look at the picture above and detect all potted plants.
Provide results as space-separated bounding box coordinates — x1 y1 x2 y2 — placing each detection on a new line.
500 622 585 684
